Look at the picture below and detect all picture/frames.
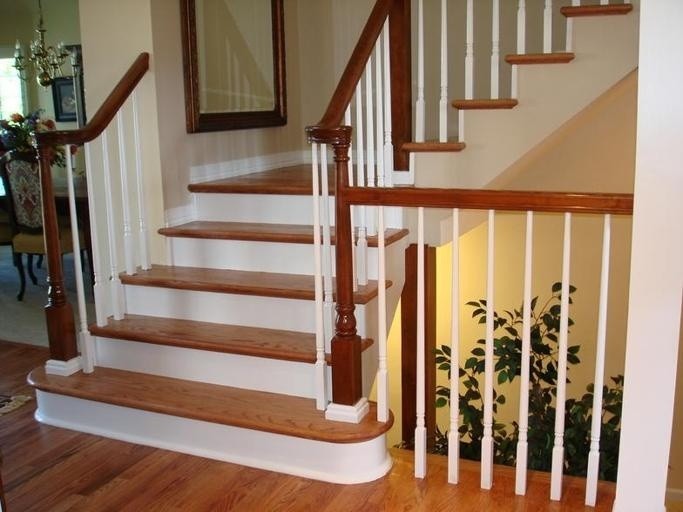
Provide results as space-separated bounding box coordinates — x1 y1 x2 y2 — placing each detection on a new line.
51 76 77 121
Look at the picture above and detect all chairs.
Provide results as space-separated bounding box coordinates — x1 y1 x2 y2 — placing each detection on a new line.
0 151 95 300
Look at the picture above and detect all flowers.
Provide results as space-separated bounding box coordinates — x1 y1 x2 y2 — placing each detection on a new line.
0 109 77 167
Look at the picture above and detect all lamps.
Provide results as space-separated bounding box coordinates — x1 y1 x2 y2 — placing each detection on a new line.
12 0 73 86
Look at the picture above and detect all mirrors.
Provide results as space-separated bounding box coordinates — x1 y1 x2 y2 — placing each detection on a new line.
179 0 288 133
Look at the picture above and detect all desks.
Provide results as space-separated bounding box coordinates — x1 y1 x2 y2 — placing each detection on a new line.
0 186 90 243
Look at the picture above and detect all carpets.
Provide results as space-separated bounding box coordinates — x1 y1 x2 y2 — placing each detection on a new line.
0 393 33 415
